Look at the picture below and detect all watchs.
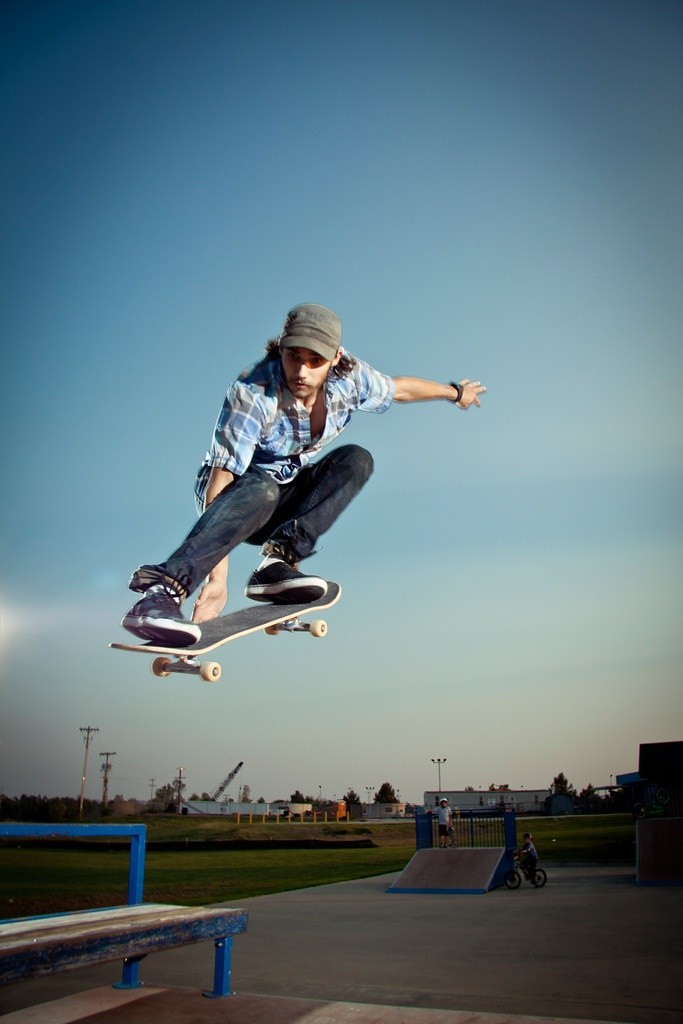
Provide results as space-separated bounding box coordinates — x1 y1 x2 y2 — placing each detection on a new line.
448 381 464 403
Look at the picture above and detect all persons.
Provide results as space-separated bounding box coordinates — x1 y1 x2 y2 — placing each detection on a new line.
425 798 452 848
122 304 487 648
517 833 539 889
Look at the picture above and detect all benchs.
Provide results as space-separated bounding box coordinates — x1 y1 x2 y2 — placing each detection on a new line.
0 903 250 999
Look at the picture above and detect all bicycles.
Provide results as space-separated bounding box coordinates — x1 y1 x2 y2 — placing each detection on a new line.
505 848 547 890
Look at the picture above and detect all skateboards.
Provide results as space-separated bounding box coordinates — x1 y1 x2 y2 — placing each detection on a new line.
107 580 342 684
448 826 458 848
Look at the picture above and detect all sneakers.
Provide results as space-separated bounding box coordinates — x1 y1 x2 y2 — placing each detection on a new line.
245 546 328 605
121 574 202 647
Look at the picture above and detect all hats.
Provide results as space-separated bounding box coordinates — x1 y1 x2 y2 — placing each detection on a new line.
279 303 341 361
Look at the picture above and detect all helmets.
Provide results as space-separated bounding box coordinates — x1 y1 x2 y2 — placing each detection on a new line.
440 798 448 803
523 834 533 839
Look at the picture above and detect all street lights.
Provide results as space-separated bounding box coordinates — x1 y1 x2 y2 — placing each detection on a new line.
432 758 447 792
365 786 375 818
